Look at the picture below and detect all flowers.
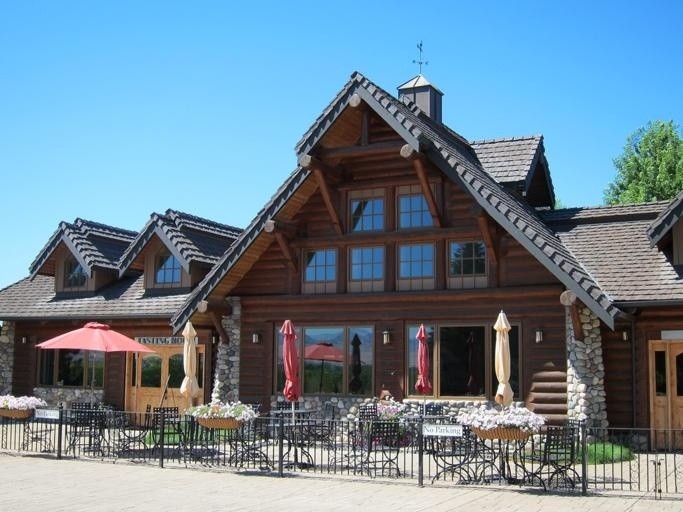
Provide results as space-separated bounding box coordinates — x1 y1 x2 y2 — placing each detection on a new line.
0 394 48 411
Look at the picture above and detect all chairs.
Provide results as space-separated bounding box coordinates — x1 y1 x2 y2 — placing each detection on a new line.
336 403 584 490
20 403 151 464
151 403 337 472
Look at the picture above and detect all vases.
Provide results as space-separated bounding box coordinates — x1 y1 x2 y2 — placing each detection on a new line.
0 407 32 419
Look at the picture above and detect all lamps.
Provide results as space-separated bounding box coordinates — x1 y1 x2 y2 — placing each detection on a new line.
382 328 391 344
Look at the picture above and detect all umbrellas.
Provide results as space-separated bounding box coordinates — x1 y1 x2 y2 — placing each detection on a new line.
304 342 344 394
350 333 362 392
412 323 432 420
466 331 481 397
493 309 515 411
34 322 156 412
179 319 200 413
279 319 302 423
